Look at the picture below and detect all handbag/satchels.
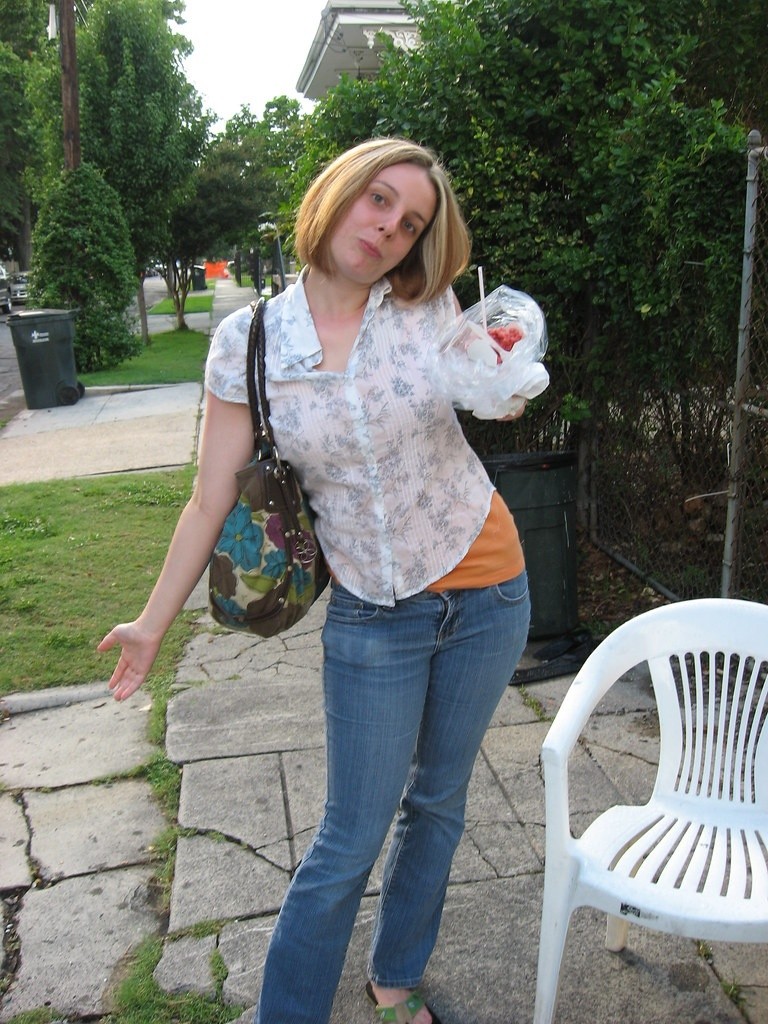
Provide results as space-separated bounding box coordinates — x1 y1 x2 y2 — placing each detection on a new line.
209 296 332 639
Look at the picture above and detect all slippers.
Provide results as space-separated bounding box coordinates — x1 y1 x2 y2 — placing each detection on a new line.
365 982 441 1024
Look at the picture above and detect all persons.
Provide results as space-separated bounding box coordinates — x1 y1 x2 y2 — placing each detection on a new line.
97 137 530 1024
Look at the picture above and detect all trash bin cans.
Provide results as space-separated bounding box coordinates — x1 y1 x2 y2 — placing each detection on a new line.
191 266 207 290
5 308 84 409
480 447 579 638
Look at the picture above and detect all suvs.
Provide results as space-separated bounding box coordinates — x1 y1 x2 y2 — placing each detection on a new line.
0 262 12 313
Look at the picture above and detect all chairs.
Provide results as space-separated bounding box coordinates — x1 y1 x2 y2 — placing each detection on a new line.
531 597 768 1022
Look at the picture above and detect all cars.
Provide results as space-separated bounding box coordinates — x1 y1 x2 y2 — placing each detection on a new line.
145 260 167 277
9 271 35 304
